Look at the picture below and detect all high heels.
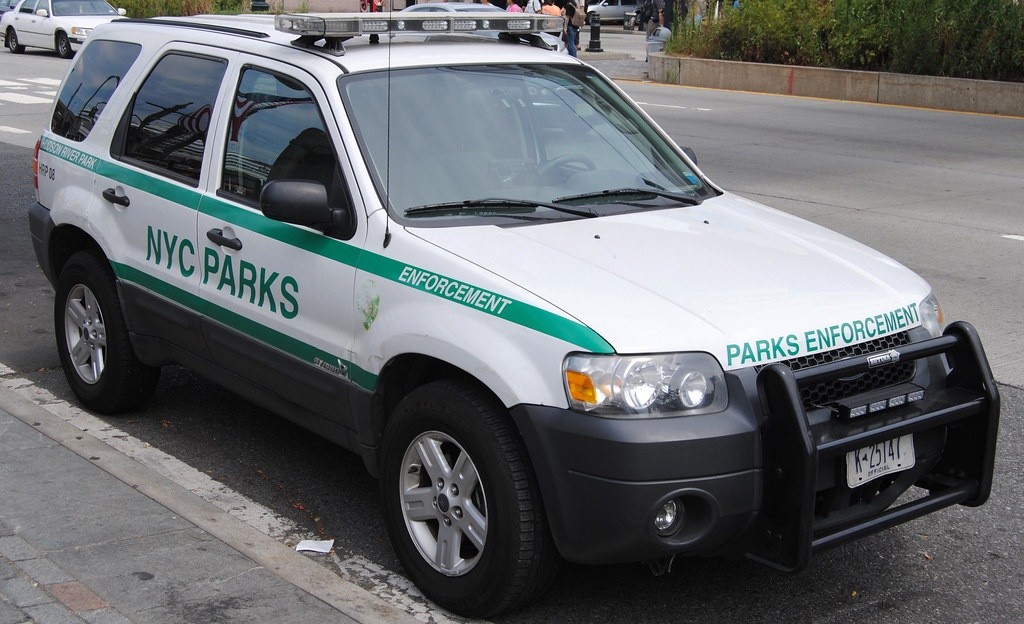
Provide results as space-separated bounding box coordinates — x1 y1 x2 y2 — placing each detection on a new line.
576 45 581 51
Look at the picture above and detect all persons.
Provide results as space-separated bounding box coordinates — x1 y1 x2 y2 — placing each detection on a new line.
646 0 670 62
480 0 579 58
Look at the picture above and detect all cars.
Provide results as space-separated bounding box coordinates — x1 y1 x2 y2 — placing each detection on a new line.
382 0 570 56
584 0 654 27
0 0 130 60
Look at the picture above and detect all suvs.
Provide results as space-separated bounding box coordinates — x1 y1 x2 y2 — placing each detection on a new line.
25 7 1002 621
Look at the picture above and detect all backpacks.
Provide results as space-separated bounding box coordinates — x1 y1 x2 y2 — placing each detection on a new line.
569 2 586 27
